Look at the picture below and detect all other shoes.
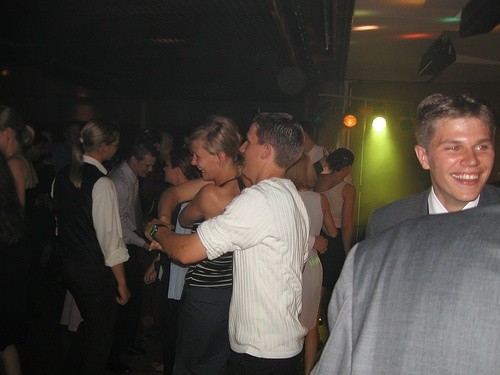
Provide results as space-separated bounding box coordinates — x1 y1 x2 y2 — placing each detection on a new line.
151 360 163 371
142 318 155 344
105 359 132 375
115 345 146 356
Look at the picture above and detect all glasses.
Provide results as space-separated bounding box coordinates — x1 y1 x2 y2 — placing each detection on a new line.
107 141 121 150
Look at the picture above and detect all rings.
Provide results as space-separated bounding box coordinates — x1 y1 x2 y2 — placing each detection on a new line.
322 247 325 249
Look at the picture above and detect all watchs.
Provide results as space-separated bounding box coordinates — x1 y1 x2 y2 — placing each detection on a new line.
150 224 169 241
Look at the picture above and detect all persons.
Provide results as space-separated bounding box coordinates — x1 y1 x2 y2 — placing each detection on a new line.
365 93 500 239
50 119 129 375
144 112 307 375
0 105 357 375
310 203 500 375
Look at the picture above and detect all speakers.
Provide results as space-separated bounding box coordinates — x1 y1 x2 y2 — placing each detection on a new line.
417 39 456 78
460 0 500 38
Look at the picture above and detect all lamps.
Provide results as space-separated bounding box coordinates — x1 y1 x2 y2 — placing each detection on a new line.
310 102 341 128
341 101 363 130
